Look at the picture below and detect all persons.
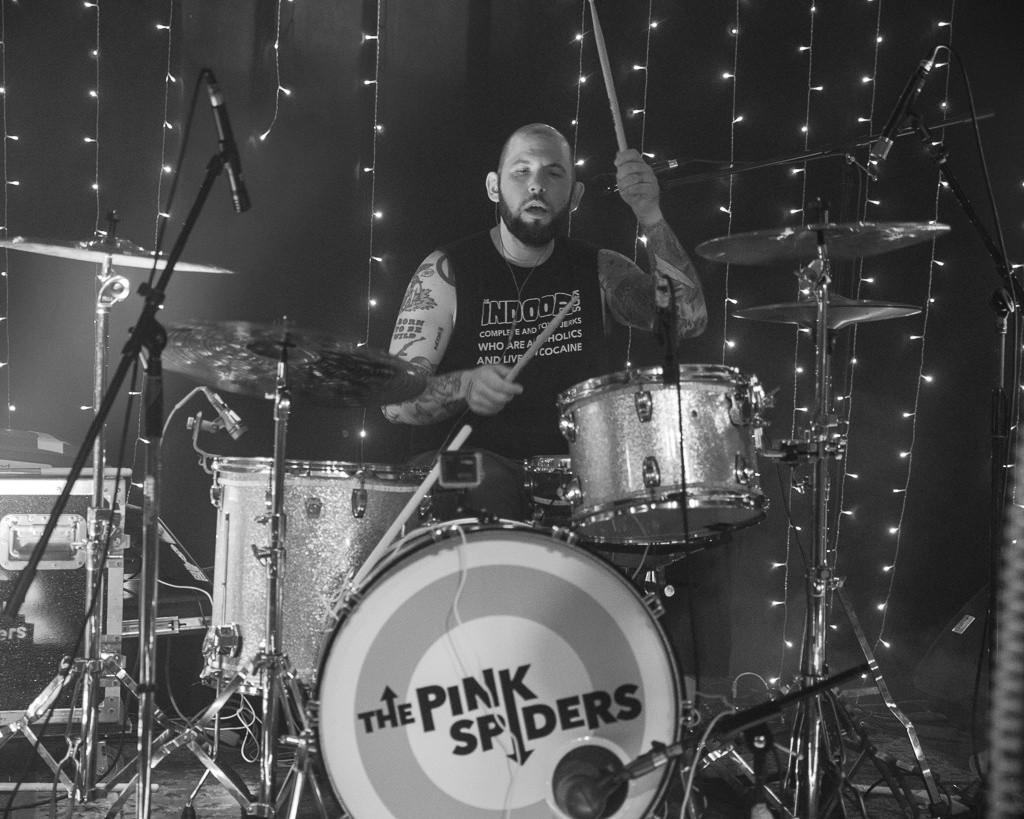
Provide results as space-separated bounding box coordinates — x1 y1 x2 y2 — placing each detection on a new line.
379 123 708 528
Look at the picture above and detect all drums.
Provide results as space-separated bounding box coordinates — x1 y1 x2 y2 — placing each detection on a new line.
197 454 436 702
523 455 678 566
555 363 769 546
299 518 688 819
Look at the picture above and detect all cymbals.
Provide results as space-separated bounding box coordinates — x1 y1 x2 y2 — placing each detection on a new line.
137 319 428 409
694 221 951 266
731 292 924 330
0 232 242 276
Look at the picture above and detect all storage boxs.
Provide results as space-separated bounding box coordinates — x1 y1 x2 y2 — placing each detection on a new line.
0 466 135 725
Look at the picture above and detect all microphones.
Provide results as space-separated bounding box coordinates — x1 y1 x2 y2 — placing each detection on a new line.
870 47 938 161
201 386 250 441
204 72 251 214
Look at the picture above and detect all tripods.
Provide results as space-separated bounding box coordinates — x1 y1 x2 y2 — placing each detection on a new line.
728 231 930 819
0 254 340 819
